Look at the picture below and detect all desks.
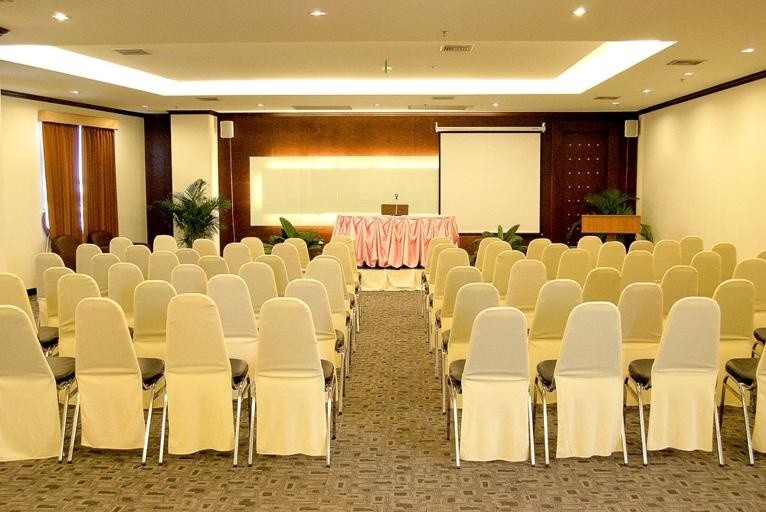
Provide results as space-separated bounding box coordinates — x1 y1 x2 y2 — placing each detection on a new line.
329 214 459 270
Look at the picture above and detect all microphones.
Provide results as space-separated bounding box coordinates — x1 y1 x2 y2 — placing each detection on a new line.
391 193 402 216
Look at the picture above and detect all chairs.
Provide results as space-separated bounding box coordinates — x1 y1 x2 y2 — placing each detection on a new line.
381 204 408 214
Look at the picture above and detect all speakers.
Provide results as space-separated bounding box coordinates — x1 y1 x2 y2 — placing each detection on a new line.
625 119 639 137
220 121 234 139
